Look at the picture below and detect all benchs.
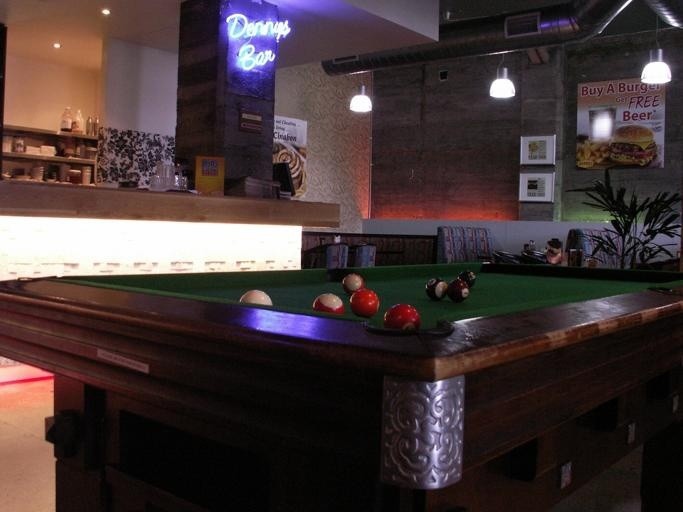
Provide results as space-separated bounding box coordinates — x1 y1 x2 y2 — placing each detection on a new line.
435 225 497 264
565 226 623 272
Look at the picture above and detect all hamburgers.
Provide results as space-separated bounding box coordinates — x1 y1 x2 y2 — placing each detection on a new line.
608 124 657 166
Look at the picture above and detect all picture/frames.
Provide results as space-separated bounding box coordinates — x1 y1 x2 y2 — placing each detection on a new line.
517 170 555 206
518 134 556 168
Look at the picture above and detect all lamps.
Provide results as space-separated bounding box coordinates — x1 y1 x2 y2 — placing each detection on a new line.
348 68 374 113
489 51 518 100
640 1 671 85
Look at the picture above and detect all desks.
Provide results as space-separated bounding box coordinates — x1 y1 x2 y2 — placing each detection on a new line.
0 260 682 510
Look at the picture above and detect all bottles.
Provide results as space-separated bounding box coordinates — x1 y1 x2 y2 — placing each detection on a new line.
62 137 97 162
58 106 99 137
28 161 94 186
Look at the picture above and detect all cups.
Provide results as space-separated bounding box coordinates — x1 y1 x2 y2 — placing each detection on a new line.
150 162 175 190
1 133 25 154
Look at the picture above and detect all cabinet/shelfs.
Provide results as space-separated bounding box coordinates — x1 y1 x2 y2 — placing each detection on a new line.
0 123 101 189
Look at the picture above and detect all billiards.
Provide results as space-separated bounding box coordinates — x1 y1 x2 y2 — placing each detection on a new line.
383 303 421 331
238 289 274 306
342 273 381 318
425 270 476 302
312 293 346 314
